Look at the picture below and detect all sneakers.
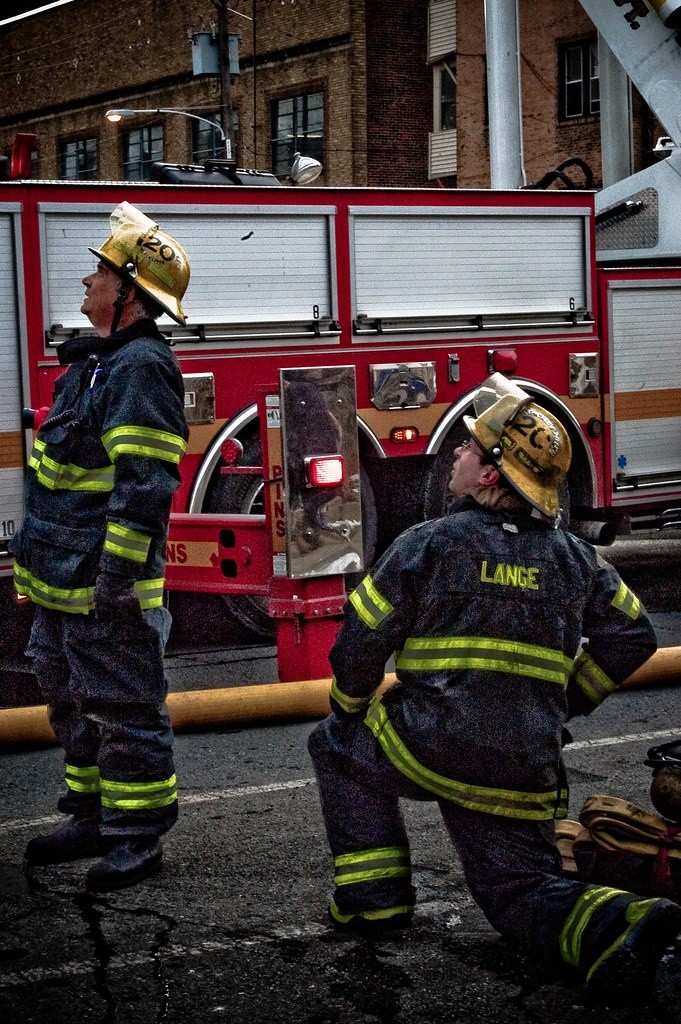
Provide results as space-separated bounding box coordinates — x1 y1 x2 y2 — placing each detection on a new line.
586 898 681 996
329 884 416 932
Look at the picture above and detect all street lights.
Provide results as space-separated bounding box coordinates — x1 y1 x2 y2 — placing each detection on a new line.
105 108 232 160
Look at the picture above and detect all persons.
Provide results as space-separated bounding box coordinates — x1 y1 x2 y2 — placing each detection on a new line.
10 199 189 892
309 373 681 1024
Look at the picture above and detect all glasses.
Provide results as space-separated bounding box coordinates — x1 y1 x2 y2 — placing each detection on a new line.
461 440 483 459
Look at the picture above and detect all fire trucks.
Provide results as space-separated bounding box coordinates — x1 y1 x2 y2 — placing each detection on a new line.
0 0 681 644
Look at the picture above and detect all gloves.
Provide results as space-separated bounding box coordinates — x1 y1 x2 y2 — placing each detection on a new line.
324 710 353 761
90 573 144 641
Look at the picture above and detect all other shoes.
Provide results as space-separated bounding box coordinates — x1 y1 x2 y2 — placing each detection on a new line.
88 835 163 893
23 813 110 864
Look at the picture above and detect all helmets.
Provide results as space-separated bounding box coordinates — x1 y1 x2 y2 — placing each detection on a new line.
463 372 573 518
89 201 191 327
